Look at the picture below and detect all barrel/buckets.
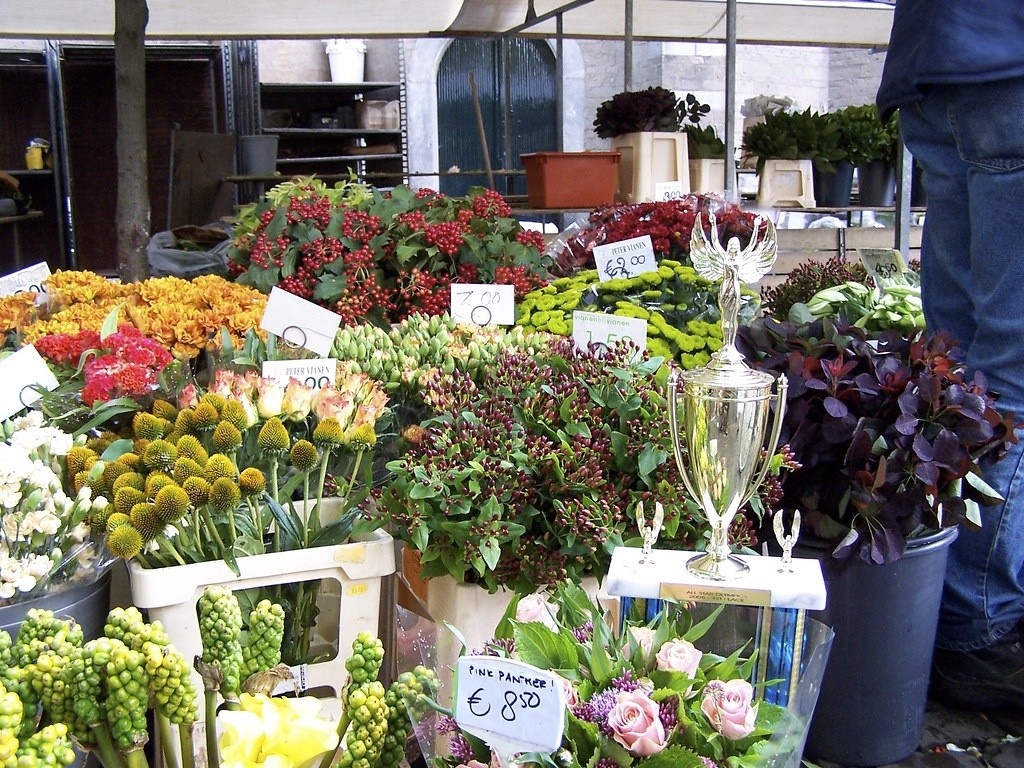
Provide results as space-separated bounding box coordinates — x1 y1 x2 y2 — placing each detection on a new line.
238 135 279 176
324 45 367 83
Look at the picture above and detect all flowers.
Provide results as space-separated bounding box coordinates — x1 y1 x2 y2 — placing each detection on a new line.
0 409 112 605
177 365 390 498
215 692 340 768
414 576 806 768
0 269 277 418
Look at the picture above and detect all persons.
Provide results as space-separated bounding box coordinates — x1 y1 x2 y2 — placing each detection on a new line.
875 1 1024 740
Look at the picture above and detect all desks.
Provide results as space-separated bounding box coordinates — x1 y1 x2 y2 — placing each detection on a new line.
0 210 44 269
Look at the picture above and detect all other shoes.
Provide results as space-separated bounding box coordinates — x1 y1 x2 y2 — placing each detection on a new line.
935 635 1024 707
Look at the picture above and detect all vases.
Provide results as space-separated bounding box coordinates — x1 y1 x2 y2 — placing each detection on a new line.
241 134 279 176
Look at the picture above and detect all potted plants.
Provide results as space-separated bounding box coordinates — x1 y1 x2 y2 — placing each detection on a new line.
735 315 1024 768
738 105 830 209
592 85 711 205
848 104 900 207
813 109 854 207
681 122 738 194
519 149 622 210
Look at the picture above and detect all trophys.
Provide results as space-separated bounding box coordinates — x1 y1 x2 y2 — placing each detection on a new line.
600 211 830 709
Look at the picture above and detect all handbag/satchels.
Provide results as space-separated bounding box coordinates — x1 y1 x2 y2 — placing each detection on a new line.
146 226 236 281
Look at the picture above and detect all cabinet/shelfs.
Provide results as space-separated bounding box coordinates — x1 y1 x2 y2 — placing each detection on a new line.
259 79 402 191
